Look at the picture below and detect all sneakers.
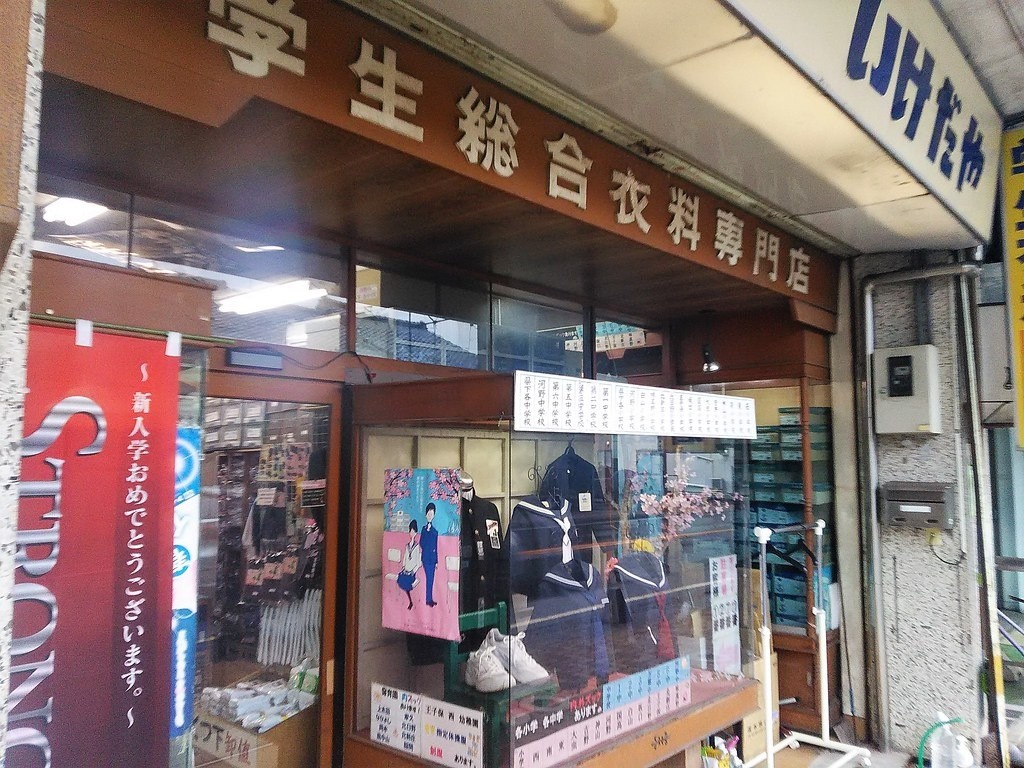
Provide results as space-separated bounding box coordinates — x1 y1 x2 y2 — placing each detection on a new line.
465 646 517 692
479 628 550 684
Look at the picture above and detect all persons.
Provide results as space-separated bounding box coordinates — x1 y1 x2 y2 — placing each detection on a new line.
407 468 519 666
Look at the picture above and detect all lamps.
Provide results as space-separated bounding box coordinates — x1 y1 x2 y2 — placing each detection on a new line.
699 309 722 374
214 275 336 316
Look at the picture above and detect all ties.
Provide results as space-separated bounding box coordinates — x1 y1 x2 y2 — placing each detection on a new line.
554 516 574 564
654 589 675 662
580 591 611 680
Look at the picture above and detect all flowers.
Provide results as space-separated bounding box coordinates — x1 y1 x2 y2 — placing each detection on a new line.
631 453 745 552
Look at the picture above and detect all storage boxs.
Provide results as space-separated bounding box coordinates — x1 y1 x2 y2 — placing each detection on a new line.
692 609 780 764
193 668 320 767
715 407 837 628
356 269 487 323
203 397 313 452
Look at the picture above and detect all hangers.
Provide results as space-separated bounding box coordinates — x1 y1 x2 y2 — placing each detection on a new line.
563 433 578 462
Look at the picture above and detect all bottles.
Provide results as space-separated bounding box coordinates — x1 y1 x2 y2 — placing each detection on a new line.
931 714 954 767
954 736 973 767
